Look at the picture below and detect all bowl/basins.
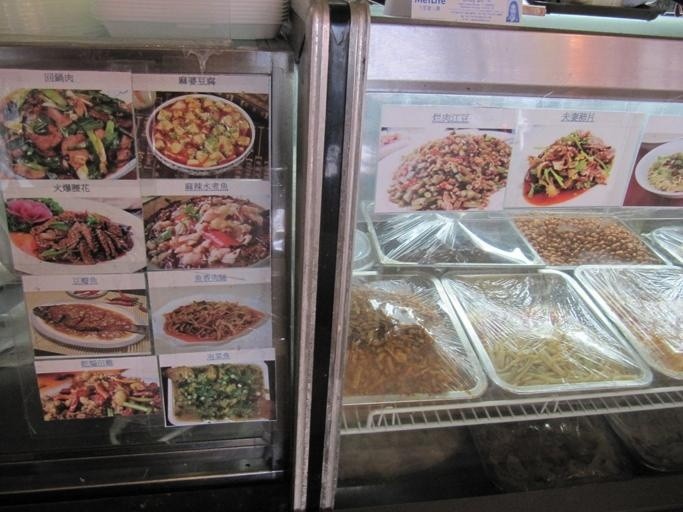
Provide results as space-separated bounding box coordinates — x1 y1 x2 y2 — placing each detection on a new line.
145 92 256 175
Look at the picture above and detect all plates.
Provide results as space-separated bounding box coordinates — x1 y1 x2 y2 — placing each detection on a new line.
4 88 137 179
7 198 146 274
161 360 273 426
141 198 270 270
30 301 148 349
151 291 271 347
375 131 515 212
42 376 161 415
634 136 682 199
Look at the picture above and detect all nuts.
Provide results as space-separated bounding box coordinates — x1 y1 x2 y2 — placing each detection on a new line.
515 210 663 267
